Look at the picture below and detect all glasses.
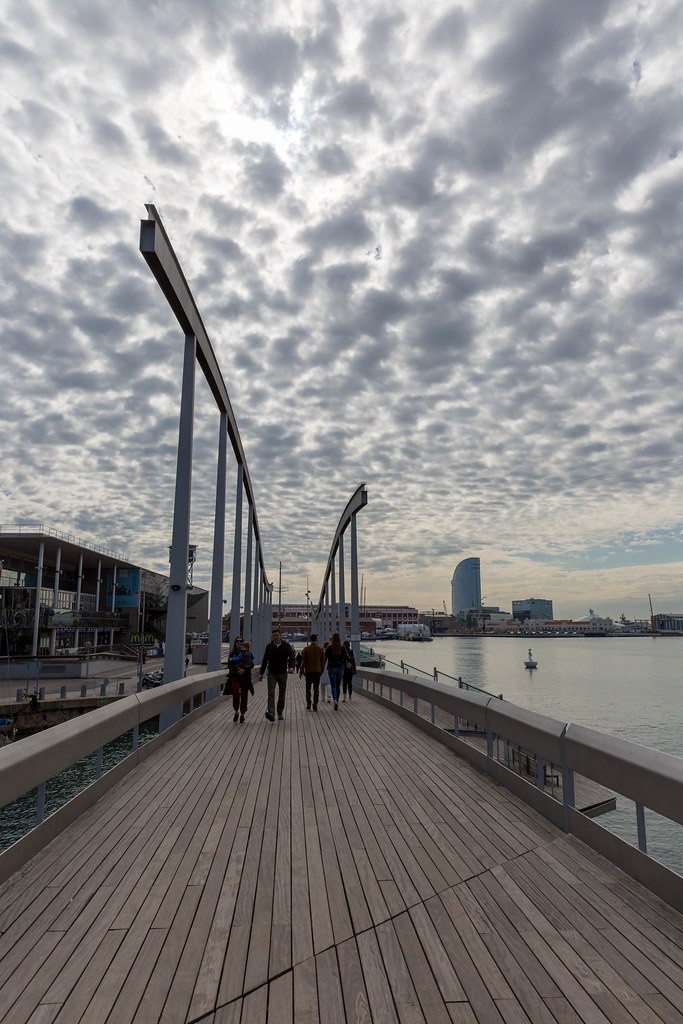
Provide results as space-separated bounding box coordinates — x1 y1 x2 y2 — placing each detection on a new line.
236 639 244 642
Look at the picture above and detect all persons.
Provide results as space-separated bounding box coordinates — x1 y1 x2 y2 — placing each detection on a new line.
185 657 189 668
299 633 326 711
295 632 357 711
257 629 295 722
222 636 254 723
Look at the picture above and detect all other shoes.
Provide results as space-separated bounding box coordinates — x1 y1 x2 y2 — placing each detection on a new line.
342 700 346 702
265 712 275 722
326 697 332 702
334 702 339 711
233 713 239 722
313 705 317 711
278 713 283 720
306 706 311 709
239 715 245 723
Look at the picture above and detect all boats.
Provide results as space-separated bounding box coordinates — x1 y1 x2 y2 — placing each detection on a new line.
524 661 537 667
281 628 434 643
